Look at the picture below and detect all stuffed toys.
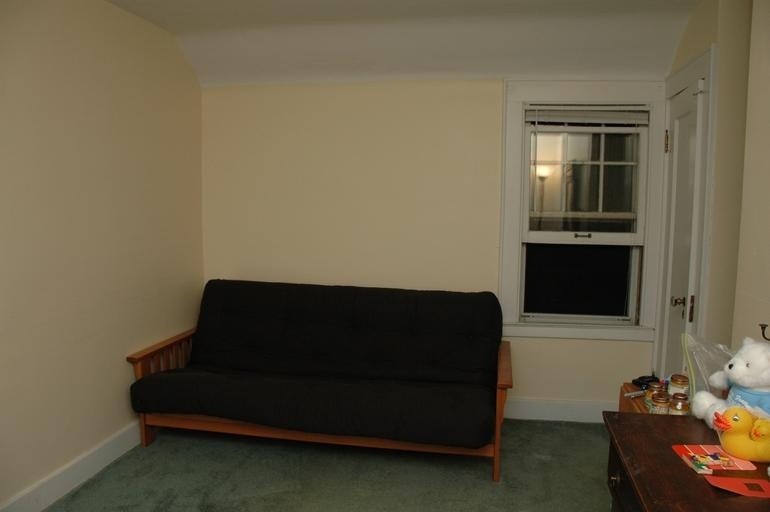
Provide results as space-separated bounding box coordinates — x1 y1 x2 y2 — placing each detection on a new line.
689 331 770 430
711 408 770 463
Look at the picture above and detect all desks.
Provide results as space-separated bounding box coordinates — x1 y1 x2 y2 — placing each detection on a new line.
596 410 770 512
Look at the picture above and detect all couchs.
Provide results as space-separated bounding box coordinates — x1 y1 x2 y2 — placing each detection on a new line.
124 269 520 483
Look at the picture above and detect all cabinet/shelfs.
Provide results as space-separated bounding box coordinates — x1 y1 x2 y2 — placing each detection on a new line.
615 379 693 415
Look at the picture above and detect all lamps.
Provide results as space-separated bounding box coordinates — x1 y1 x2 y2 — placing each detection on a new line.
530 161 557 228
